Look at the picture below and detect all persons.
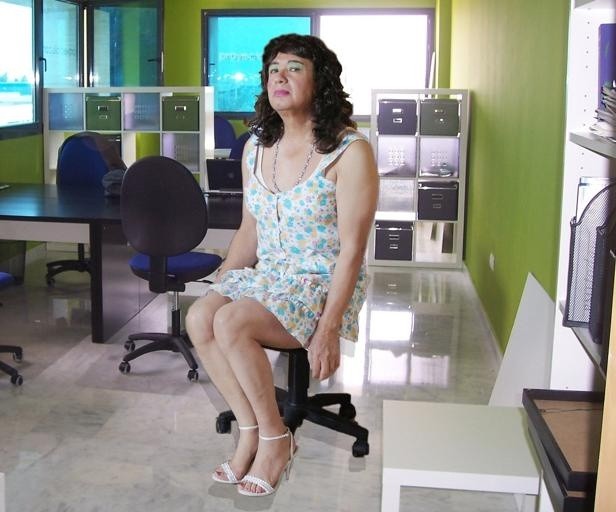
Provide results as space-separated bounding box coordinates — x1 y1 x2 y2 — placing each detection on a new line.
185 31 381 496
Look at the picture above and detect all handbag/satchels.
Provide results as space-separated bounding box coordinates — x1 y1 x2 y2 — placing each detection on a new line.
102 169 124 197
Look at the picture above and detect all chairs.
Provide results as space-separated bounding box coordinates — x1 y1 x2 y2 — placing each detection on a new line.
45 131 128 287
119 155 223 382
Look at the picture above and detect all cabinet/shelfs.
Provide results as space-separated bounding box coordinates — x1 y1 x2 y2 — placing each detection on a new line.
367 88 471 268
536 0 616 511
42 86 216 253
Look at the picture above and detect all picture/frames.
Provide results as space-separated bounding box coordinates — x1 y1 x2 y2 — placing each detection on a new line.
521 388 605 511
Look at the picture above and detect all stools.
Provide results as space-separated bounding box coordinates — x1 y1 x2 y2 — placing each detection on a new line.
214 346 370 458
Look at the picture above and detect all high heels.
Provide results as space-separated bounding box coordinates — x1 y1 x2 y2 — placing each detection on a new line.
237 427 299 497
211 424 259 484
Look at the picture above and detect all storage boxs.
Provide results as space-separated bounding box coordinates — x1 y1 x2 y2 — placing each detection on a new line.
376 98 459 136
86 95 121 131
373 219 413 262
161 94 200 131
418 183 458 220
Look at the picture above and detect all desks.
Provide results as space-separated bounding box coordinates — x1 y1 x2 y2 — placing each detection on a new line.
377 399 541 512
0 180 242 343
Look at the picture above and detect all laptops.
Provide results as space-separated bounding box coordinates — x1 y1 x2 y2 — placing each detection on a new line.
206 158 242 191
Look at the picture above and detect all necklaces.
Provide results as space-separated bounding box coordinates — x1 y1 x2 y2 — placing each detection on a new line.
271 137 314 193
